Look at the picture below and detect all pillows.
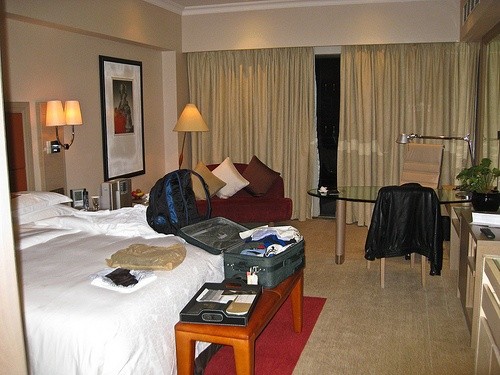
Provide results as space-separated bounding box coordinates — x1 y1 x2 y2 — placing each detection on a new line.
191 161 226 200
242 155 280 198
11 190 79 226
212 157 250 199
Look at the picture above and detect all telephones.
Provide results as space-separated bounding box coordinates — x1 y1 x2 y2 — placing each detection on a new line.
70 188 88 208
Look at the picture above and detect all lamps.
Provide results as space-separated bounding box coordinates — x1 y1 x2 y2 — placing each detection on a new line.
396 133 475 200
46 100 83 154
173 104 210 169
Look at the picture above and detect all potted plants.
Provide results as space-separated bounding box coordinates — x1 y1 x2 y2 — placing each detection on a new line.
456 158 500 212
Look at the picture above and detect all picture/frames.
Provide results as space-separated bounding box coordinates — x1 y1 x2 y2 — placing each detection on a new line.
98 54 145 182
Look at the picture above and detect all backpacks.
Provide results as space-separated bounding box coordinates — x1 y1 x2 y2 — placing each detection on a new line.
146 169 212 235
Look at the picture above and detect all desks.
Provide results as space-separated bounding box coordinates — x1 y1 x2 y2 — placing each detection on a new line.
307 185 471 265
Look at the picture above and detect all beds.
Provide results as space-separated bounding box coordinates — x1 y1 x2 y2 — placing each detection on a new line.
11 101 225 375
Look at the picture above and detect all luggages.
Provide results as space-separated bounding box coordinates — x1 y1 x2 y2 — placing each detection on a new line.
178 216 305 288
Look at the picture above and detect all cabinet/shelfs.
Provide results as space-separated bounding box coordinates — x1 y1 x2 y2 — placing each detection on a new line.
441 184 500 375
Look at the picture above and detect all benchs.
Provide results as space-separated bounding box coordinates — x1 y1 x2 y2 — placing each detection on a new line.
195 163 292 227
174 266 303 375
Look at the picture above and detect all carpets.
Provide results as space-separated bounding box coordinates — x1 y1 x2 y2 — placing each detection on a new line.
202 294 327 375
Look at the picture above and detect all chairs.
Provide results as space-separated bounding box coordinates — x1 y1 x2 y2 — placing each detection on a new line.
364 143 445 289
